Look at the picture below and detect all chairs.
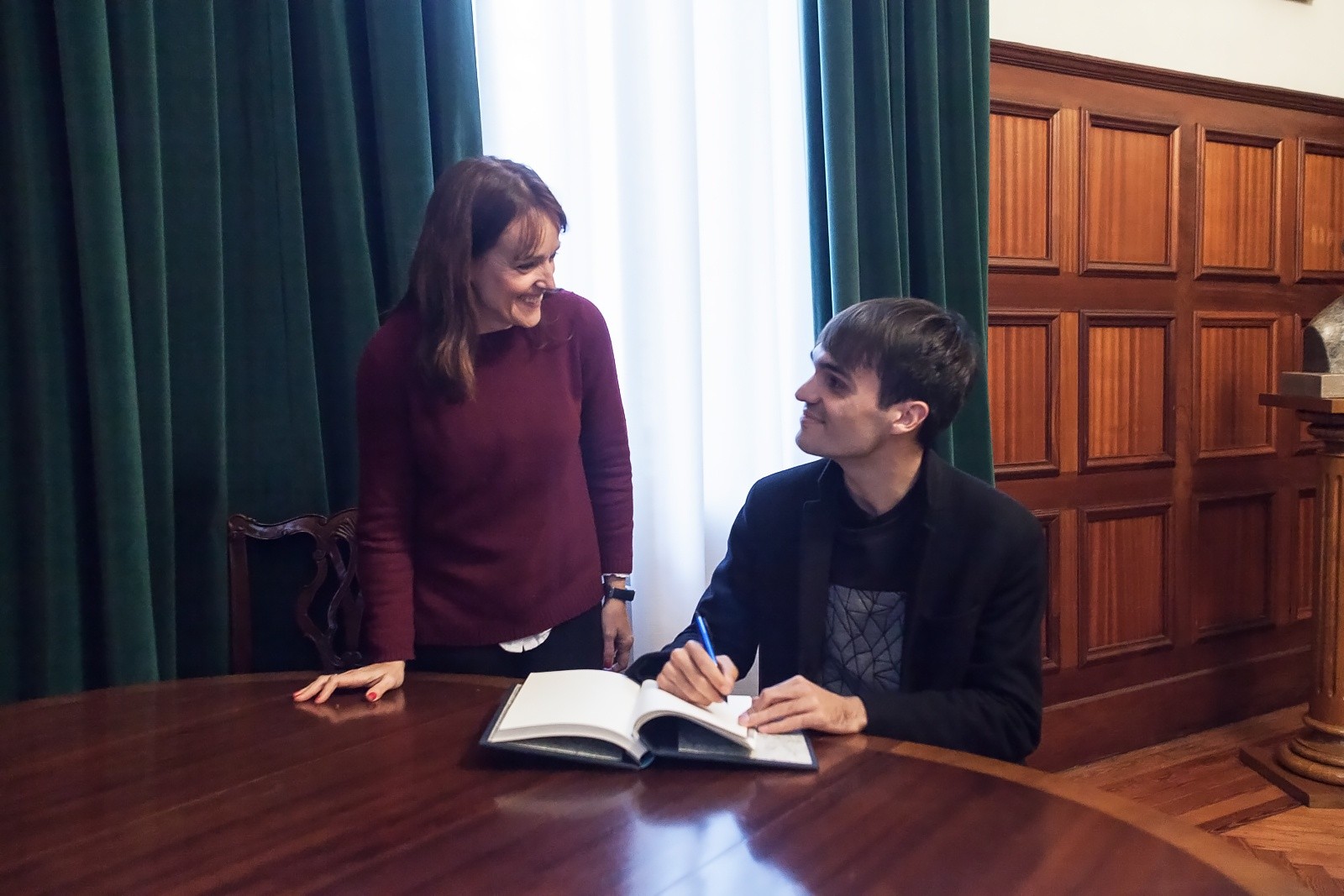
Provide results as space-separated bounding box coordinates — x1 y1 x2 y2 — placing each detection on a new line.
226 506 362 669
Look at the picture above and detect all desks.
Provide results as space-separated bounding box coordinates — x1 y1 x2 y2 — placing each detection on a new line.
0 671 1312 896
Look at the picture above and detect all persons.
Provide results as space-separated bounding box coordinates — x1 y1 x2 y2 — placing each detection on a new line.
291 152 635 707
628 295 1053 772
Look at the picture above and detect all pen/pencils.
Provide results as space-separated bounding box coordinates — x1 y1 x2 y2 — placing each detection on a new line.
694 610 727 702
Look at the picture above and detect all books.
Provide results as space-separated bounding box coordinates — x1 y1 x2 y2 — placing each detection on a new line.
485 666 819 774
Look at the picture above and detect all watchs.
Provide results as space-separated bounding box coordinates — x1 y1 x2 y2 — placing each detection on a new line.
602 583 637 601
